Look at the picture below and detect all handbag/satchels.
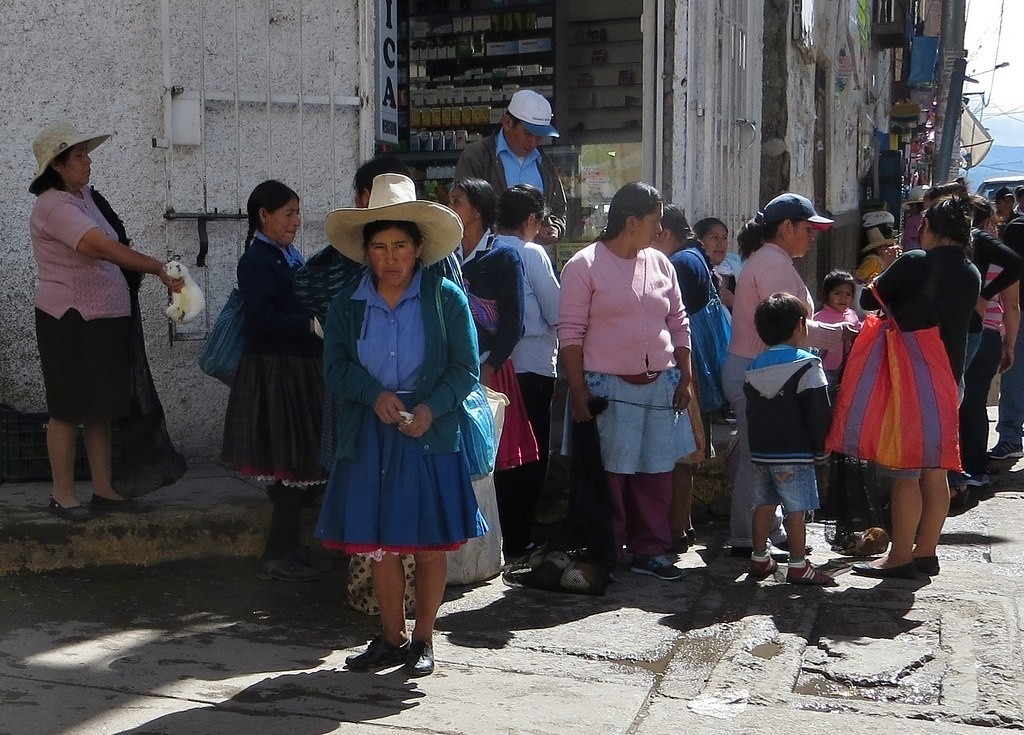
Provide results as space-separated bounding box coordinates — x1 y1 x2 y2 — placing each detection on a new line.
457 384 497 482
824 283 962 472
198 288 250 388
674 249 732 413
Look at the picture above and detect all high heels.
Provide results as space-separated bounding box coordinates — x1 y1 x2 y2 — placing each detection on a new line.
950 484 969 511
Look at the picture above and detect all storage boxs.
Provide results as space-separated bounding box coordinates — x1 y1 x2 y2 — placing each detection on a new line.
409 13 554 154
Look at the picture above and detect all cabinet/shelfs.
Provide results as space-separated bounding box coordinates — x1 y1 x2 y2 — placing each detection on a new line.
403 0 558 153
569 15 641 132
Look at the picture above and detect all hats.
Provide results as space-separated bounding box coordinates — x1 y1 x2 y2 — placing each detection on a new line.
324 173 464 268
29 120 112 194
901 185 930 210
764 193 835 231
996 187 1014 203
860 226 899 254
507 90 560 137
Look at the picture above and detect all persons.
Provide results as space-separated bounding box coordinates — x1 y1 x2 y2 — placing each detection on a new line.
28 123 184 518
654 178 1024 584
315 174 489 676
454 90 567 247
450 180 564 555
221 158 411 578
557 184 695 579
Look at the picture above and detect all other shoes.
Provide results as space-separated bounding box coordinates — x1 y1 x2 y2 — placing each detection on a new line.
678 535 689 547
288 544 323 573
915 556 940 574
851 562 917 579
687 530 697 540
91 493 152 514
48 498 96 521
731 536 834 585
256 554 320 582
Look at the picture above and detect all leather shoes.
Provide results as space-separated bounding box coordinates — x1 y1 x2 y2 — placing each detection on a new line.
345 633 410 673
404 640 434 676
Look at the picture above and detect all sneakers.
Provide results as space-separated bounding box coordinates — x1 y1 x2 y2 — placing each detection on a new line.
988 441 1023 459
966 473 990 486
630 554 685 580
